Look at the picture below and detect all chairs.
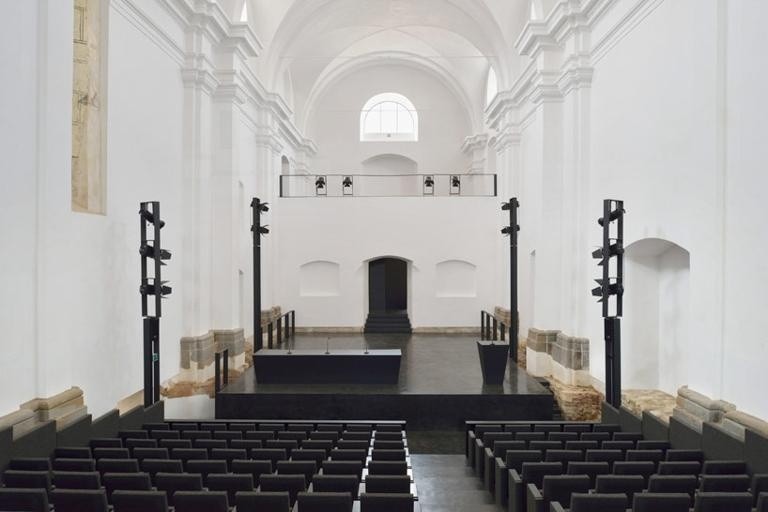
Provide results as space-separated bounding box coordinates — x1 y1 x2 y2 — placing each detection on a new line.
468 425 768 512
0 422 418 512
